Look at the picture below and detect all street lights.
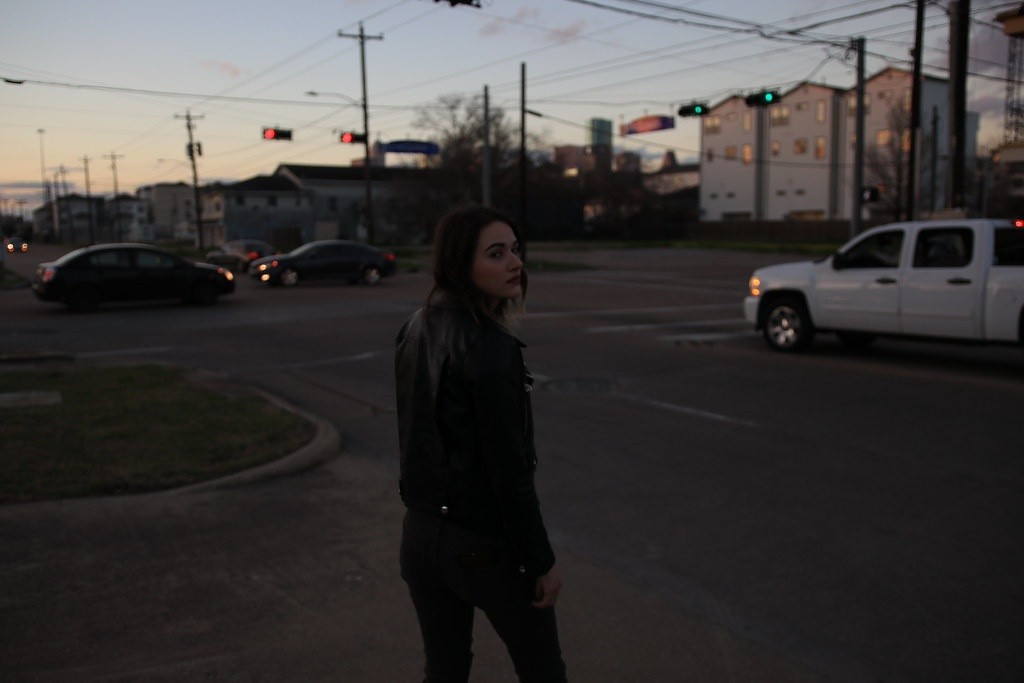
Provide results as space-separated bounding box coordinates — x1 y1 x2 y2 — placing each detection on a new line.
306 89 375 245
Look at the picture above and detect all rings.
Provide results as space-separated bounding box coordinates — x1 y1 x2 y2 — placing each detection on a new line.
544 596 552 600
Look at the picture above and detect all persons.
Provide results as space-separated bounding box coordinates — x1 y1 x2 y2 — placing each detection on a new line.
396 204 570 683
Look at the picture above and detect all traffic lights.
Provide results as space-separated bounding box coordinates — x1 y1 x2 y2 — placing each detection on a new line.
340 131 368 143
678 103 710 116
745 89 780 108
262 127 294 141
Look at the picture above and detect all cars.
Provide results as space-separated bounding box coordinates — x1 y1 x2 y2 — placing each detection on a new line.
205 238 283 269
33 242 236 313
248 240 398 288
8 238 28 254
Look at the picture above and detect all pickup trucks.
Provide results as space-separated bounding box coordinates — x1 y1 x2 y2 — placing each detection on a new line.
745 219 1023 355
